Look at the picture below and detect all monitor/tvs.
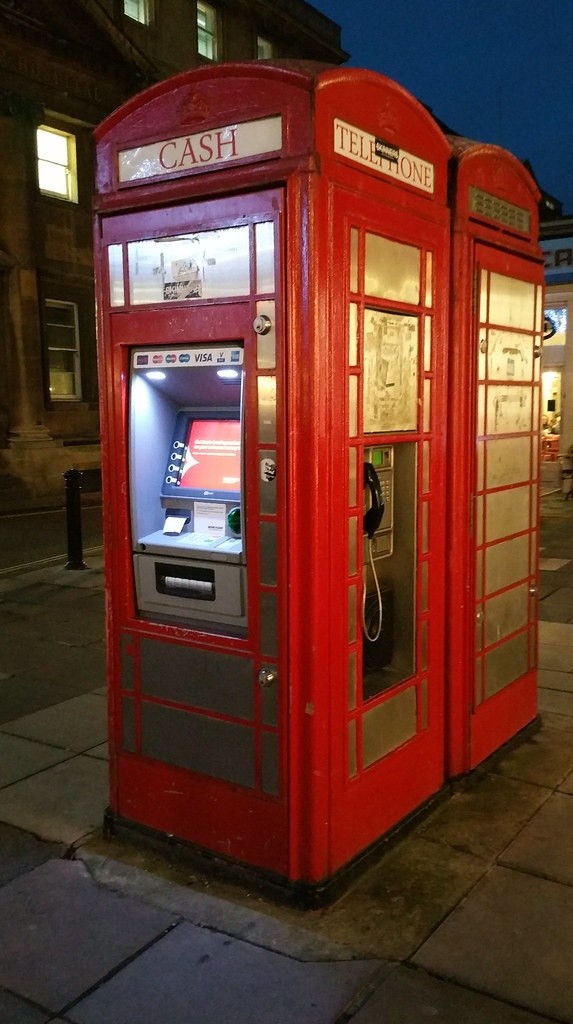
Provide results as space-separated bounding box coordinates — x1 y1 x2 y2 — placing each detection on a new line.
160 407 241 514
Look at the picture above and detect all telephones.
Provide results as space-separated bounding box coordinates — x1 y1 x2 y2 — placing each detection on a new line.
363 444 394 564
